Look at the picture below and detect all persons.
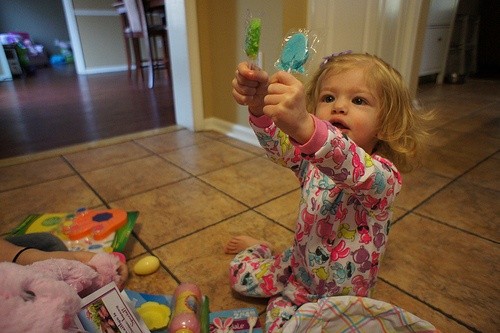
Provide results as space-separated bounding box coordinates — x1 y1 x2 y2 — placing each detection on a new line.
0 239 128 299
224 50 440 333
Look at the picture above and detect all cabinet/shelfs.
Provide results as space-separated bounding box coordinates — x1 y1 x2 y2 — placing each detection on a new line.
419 0 481 85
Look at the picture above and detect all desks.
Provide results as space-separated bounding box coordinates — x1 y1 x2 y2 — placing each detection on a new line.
111 0 171 81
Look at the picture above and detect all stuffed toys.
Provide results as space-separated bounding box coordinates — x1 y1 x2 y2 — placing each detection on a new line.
0 253 123 333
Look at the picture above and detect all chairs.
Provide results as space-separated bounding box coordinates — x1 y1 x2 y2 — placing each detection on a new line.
124 0 164 82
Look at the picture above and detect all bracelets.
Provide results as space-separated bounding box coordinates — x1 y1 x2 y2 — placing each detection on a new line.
12 247 34 263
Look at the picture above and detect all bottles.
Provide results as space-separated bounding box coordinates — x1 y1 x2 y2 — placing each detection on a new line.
168 283 202 333
